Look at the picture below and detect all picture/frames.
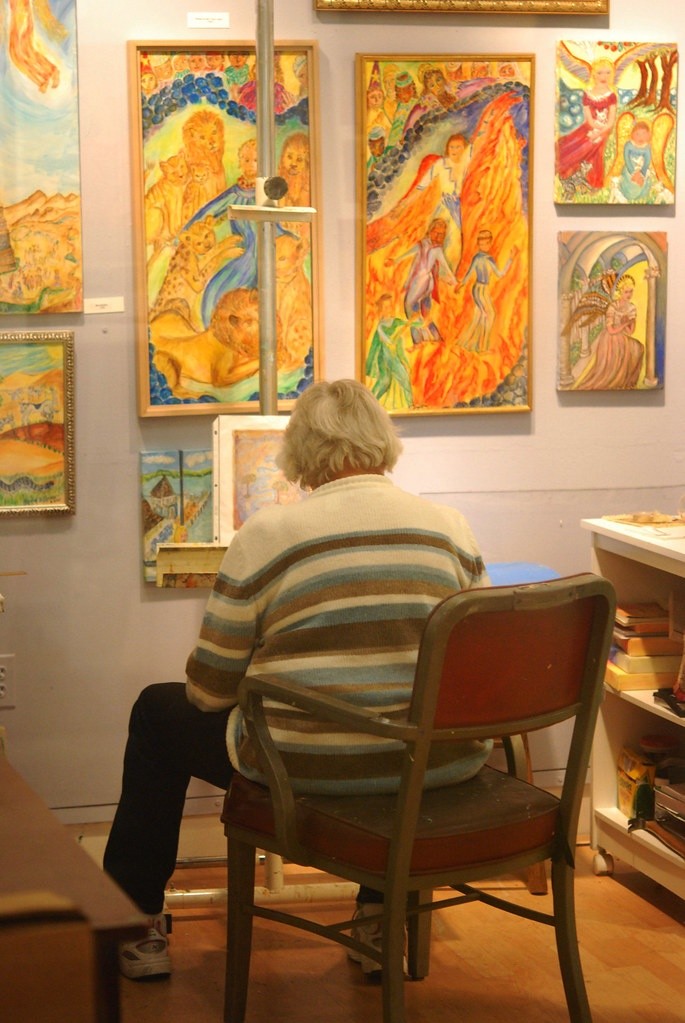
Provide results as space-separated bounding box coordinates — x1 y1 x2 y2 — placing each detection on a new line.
210 416 324 556
0 0 85 323
122 36 329 428
137 445 220 595
552 229 668 400
555 36 682 209
351 50 534 417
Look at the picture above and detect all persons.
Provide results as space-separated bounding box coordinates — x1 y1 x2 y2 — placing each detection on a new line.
81 380 494 990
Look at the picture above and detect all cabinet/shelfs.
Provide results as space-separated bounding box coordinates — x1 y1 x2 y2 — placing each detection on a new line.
575 510 684 909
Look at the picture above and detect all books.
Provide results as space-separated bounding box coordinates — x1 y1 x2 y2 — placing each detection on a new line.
604 601 685 689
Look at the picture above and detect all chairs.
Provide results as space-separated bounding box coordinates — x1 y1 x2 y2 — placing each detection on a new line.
211 570 620 1023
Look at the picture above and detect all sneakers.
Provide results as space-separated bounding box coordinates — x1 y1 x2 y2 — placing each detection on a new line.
104 914 173 981
348 907 382 977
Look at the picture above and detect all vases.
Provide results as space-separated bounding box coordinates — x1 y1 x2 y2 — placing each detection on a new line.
0 329 76 523
